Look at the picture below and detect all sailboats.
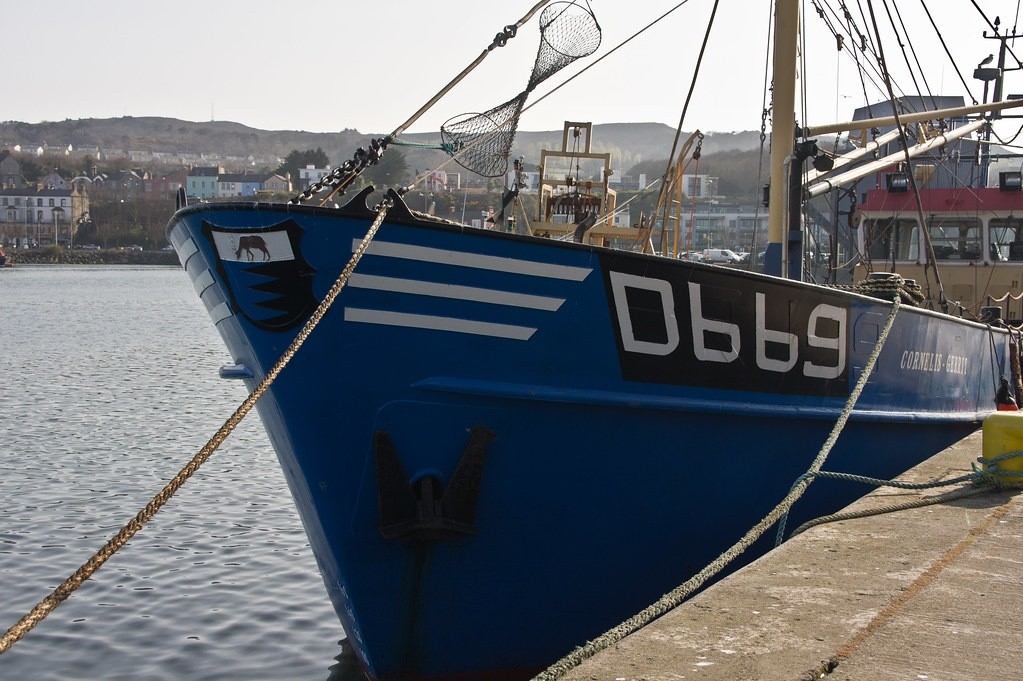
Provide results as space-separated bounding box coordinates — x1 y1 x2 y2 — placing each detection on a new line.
166 0 1023 681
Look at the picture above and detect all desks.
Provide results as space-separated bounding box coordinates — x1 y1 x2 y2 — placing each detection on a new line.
525 221 655 258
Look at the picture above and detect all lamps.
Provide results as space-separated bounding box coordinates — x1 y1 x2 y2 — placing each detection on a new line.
998 171 1022 192
886 172 907 193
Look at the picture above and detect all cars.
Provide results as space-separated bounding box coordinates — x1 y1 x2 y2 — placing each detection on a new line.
804 251 831 264
161 244 173 250
654 247 765 266
80 244 100 250
129 244 144 252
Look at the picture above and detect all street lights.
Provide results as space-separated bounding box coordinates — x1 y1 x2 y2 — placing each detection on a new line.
706 180 713 263
23 197 29 245
120 199 138 227
